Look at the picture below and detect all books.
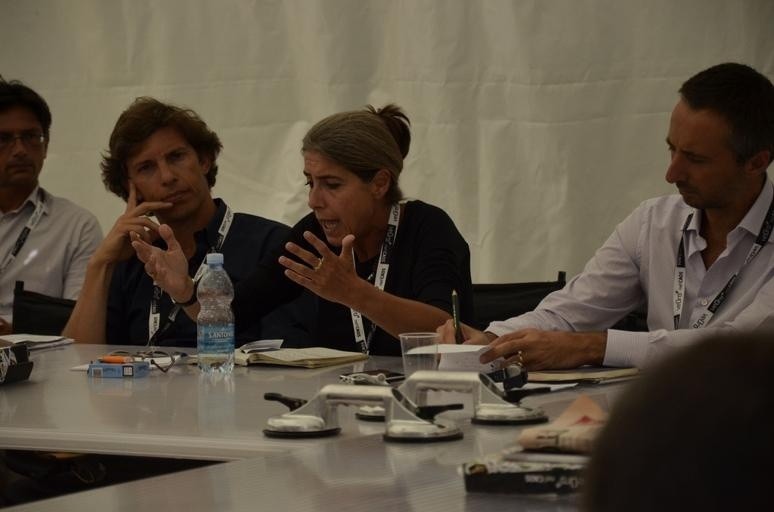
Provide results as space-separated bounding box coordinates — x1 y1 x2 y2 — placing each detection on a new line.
0 332 75 351
518 393 612 455
457 439 595 494
172 337 370 369
526 367 640 385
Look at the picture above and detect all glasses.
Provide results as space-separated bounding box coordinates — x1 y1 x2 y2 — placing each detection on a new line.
87 350 175 375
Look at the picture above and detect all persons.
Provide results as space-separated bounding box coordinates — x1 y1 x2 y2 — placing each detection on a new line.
0 76 104 336
57 94 291 348
571 324 773 512
427 62 773 374
127 103 473 357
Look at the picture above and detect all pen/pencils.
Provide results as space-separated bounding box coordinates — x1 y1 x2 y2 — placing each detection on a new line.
451 289 465 344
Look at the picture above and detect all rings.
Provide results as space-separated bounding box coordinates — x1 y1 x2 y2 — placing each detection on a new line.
313 257 322 270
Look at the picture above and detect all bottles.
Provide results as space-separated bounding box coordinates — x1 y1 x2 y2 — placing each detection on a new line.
195 253 236 376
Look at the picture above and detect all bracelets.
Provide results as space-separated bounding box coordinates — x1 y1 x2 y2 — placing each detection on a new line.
168 277 196 308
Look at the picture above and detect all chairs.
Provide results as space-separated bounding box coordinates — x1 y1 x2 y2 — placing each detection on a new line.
452 268 571 339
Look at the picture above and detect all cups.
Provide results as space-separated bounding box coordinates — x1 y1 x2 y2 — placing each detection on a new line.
398 332 442 377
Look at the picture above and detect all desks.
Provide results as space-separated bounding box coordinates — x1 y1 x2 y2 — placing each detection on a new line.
1 336 641 512
